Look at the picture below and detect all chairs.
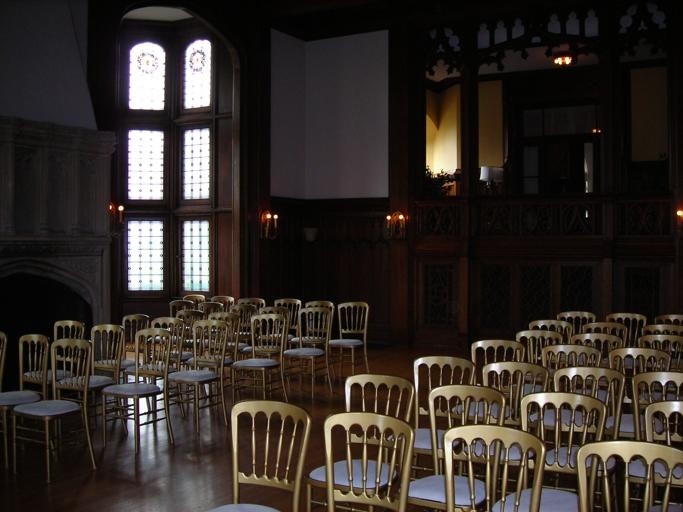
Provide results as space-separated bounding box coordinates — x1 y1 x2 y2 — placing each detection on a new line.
1 292 373 487
197 292 681 510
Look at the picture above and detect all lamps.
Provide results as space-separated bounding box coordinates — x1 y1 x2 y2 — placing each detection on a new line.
477 163 505 191
